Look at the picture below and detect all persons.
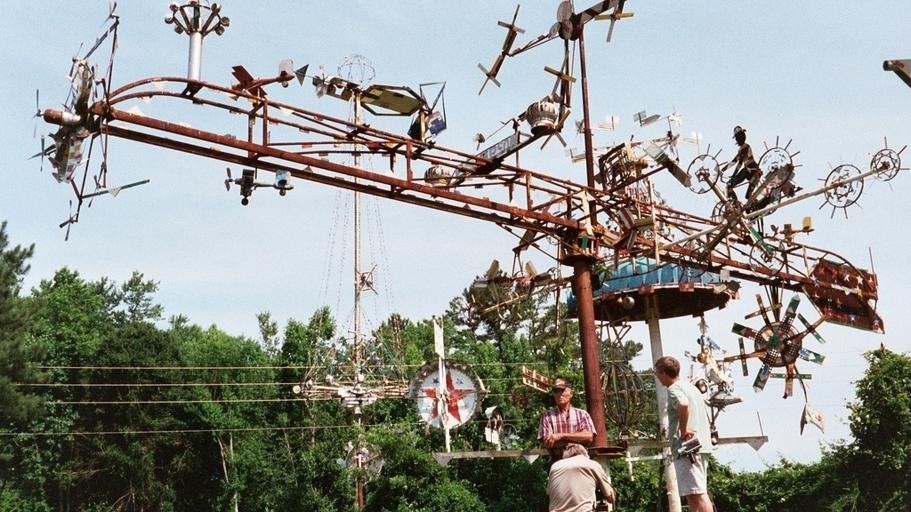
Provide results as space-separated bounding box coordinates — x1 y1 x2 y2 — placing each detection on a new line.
719 126 762 207
549 442 616 512
655 356 713 512
537 378 597 450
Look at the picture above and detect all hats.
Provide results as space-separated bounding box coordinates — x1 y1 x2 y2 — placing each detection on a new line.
552 378 572 390
